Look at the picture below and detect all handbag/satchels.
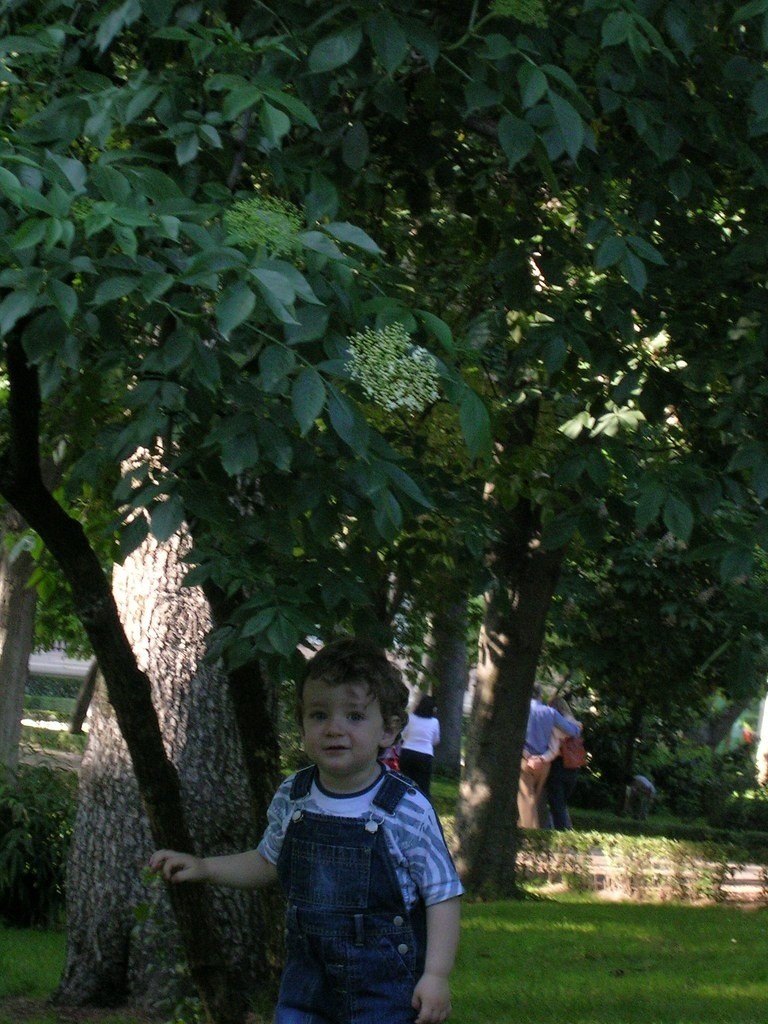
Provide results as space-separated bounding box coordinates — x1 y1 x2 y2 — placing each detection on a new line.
559 737 587 769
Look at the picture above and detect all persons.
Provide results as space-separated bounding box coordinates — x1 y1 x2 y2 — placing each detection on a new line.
623 774 656 821
517 682 584 832
149 639 465 1024
401 696 441 796
